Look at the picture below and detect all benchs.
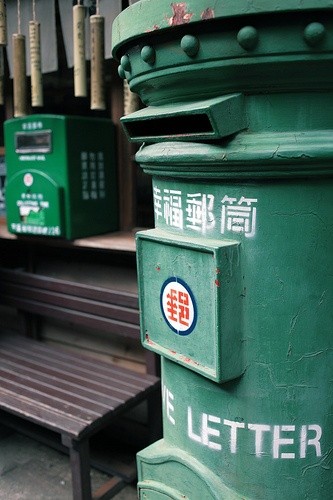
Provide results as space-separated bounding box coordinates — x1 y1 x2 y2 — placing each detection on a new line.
1 268 162 500
